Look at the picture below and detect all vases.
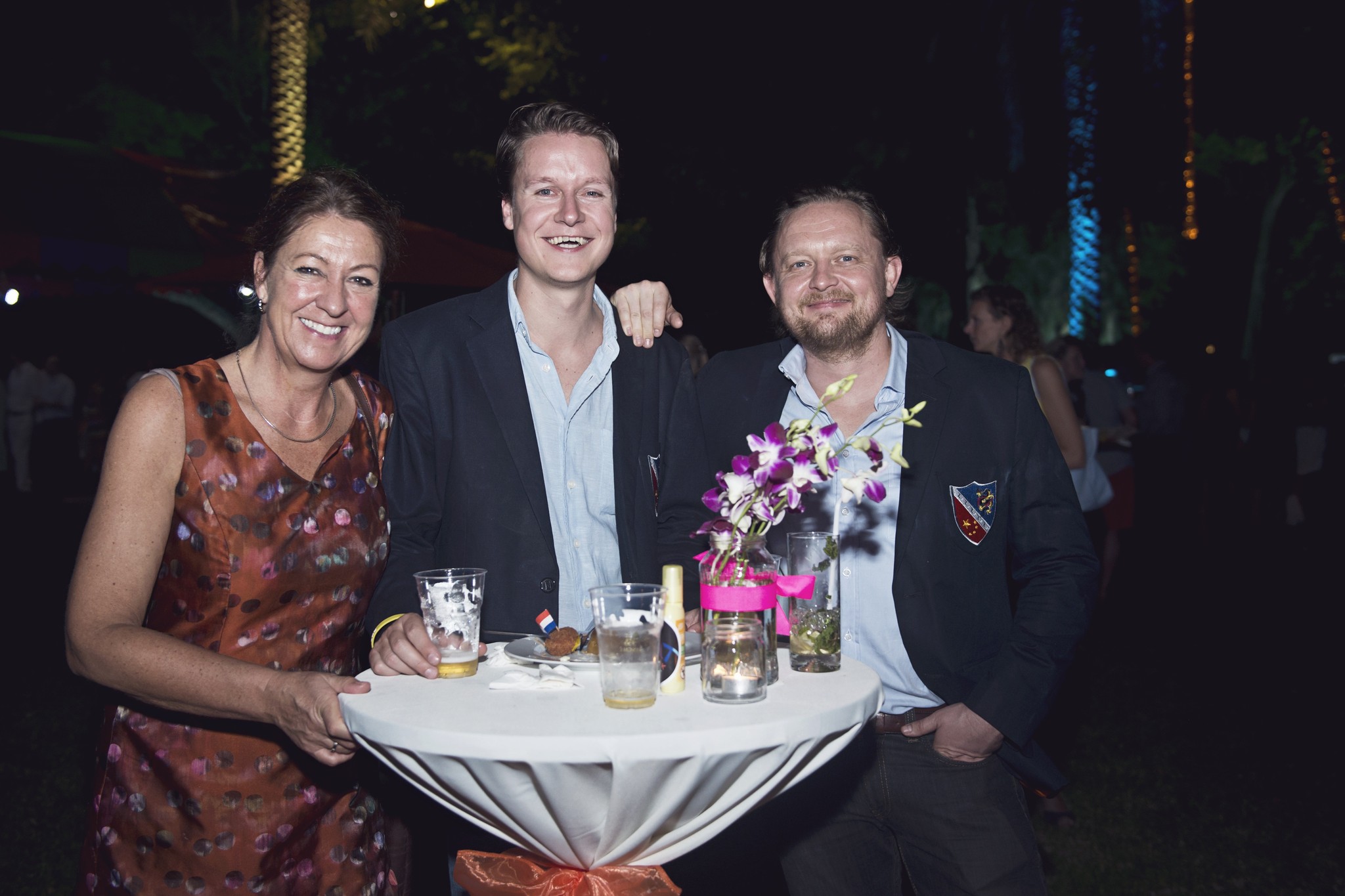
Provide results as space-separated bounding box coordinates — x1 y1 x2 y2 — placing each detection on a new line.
699 556 783 681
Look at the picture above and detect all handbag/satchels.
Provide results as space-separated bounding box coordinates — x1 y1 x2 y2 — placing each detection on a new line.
1028 355 1112 515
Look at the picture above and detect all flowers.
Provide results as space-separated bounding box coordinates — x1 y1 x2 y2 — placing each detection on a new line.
689 375 927 635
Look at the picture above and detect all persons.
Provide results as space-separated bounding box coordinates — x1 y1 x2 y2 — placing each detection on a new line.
963 284 1254 600
1 350 180 506
64 166 414 896
609 181 1094 896
357 102 712 678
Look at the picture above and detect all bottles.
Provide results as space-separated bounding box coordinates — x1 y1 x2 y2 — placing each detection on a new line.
702 618 767 704
661 565 685 693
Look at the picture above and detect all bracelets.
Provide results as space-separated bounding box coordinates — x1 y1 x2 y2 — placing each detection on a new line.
371 614 405 648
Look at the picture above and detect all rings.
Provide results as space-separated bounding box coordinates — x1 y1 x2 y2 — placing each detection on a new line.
331 742 338 752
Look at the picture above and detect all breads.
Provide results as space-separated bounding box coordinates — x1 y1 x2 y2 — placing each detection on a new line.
587 625 625 655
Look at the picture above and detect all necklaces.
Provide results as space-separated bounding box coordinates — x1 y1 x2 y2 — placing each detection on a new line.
236 348 337 442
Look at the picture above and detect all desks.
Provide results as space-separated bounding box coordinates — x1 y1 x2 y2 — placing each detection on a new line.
339 642 886 896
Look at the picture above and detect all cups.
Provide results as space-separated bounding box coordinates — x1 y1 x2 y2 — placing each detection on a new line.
788 533 841 673
588 583 668 710
771 553 782 569
414 568 488 679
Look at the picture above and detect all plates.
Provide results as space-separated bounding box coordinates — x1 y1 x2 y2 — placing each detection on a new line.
504 632 704 670
